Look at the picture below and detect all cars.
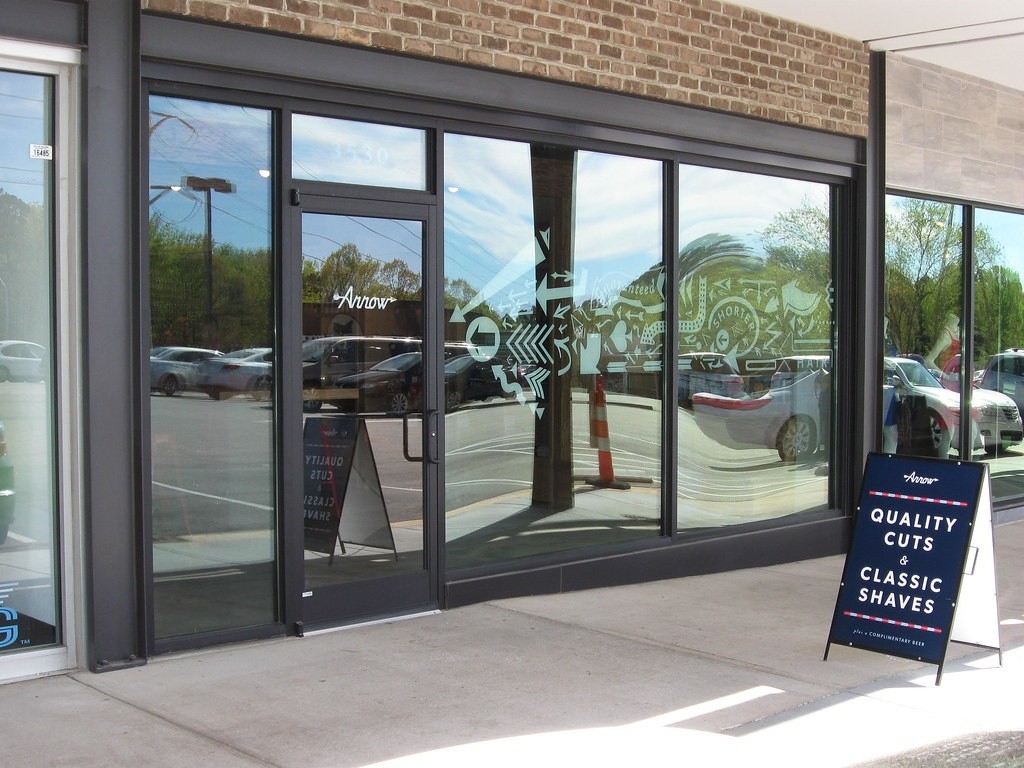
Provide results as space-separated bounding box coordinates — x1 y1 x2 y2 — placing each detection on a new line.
688 352 1024 465
150 346 235 396
303 334 422 413
334 351 426 417
0 339 50 384
516 361 536 383
196 346 272 401
445 355 522 413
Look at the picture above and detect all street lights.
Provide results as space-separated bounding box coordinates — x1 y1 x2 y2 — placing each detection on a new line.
186 176 237 350
